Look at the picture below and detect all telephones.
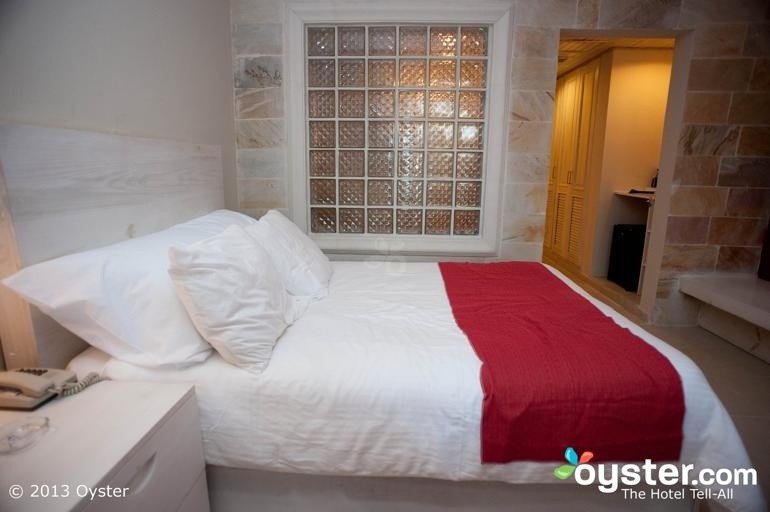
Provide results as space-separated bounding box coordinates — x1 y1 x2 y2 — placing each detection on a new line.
0 367 77 408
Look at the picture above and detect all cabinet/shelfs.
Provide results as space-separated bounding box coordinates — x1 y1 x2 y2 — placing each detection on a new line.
543 52 600 267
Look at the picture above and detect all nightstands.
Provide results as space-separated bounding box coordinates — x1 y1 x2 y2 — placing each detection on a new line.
1 378 211 512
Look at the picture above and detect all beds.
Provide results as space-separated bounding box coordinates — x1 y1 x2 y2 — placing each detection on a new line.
62 257 765 512
